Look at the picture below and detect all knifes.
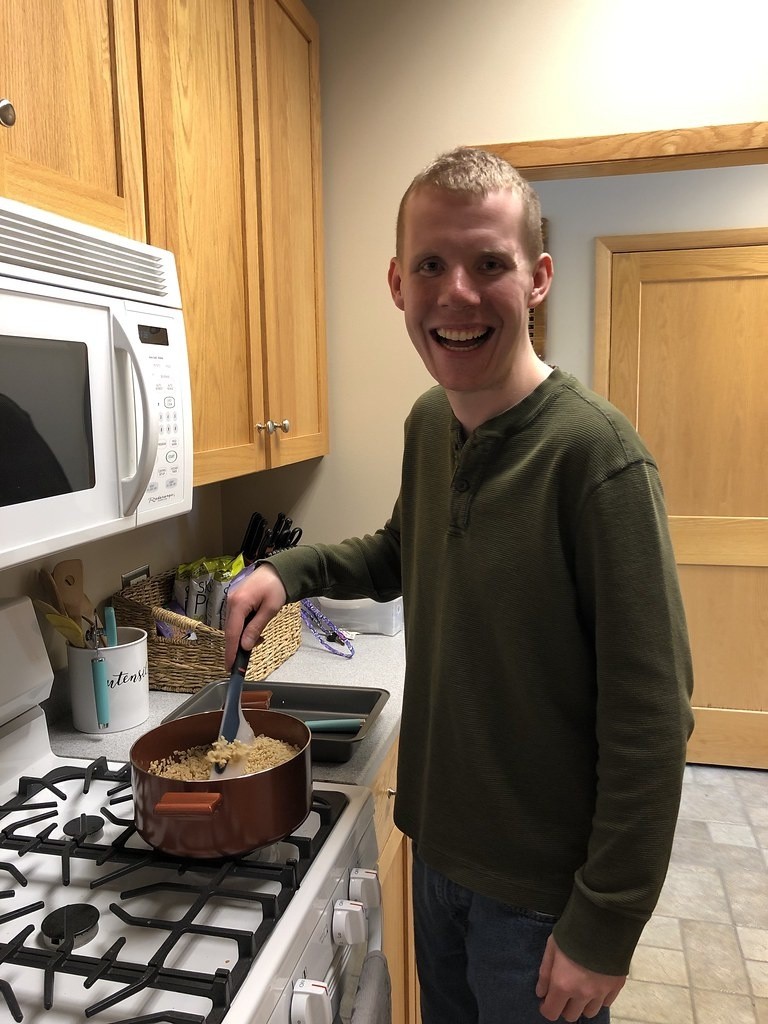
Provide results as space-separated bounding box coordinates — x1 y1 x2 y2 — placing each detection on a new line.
207 609 260 782
236 512 303 566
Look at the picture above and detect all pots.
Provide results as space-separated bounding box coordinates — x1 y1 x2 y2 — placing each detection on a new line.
129 709 314 857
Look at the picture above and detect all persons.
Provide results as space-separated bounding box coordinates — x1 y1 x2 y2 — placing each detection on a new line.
222 143 700 1024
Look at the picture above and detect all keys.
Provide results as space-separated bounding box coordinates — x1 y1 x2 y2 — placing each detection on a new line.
318 630 345 647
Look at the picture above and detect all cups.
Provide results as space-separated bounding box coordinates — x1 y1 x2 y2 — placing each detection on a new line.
66 625 151 735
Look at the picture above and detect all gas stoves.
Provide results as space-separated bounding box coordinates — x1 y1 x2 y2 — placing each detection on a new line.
1 755 384 1024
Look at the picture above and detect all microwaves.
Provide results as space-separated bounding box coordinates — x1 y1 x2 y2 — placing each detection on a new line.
0 196 194 572
